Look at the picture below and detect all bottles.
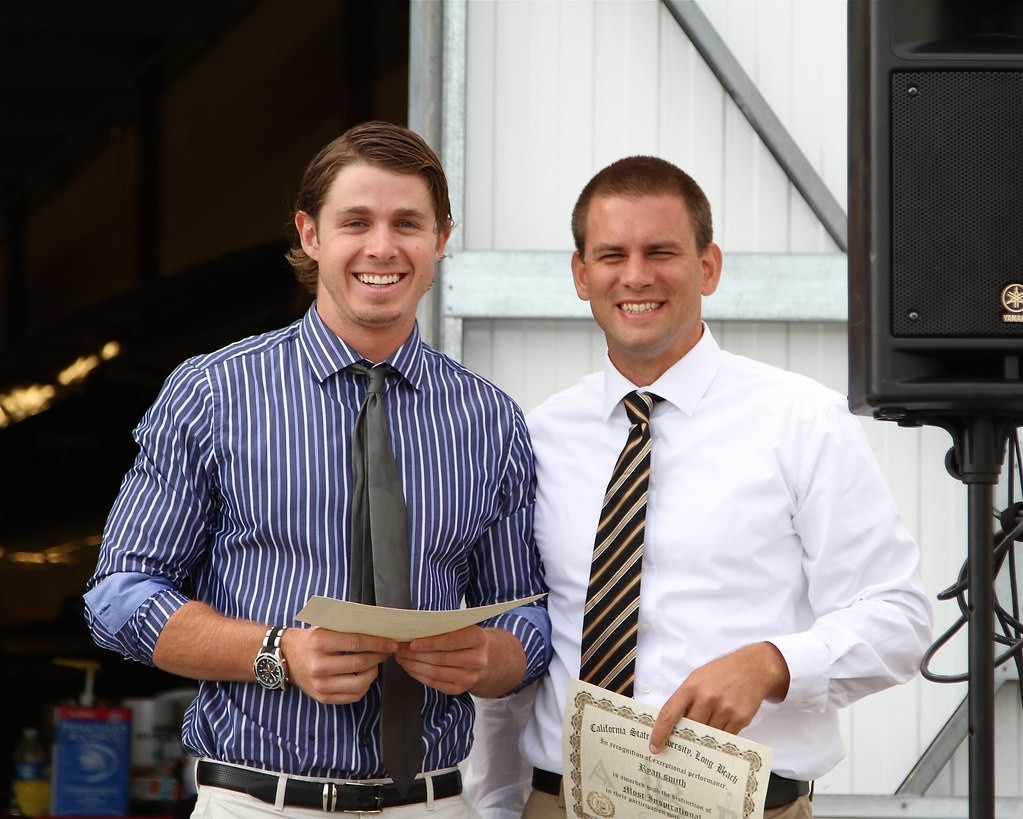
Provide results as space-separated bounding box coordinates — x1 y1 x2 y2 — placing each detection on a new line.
14 729 47 780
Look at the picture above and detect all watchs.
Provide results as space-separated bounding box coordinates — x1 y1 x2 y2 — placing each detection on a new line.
254 627 291 691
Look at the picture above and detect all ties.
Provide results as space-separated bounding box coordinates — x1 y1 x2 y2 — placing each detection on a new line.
343 362 424 787
579 390 665 697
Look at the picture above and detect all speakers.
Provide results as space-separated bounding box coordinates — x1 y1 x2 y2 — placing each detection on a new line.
847 0 1023 431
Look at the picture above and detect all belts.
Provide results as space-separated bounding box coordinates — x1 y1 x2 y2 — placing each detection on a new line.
195 760 462 814
531 768 809 810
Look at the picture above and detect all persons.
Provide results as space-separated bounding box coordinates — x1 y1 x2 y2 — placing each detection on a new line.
457 156 933 819
83 122 552 819
